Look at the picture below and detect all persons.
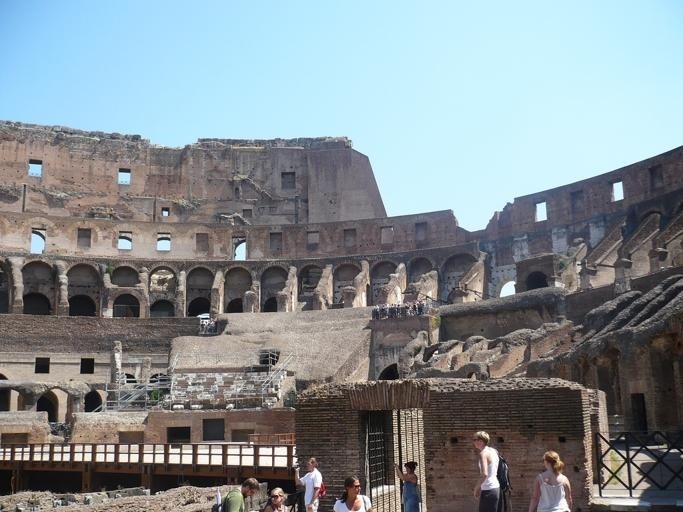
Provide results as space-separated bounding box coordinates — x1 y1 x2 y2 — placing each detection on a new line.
371 300 426 319
218 478 261 512
528 451 574 512
333 475 375 512
294 457 323 512
394 461 421 512
472 431 507 512
263 487 290 512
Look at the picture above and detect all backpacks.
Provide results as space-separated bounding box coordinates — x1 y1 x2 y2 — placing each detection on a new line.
497 456 513 494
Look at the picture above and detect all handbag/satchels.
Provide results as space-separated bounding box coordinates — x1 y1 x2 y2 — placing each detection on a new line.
318 483 326 497
